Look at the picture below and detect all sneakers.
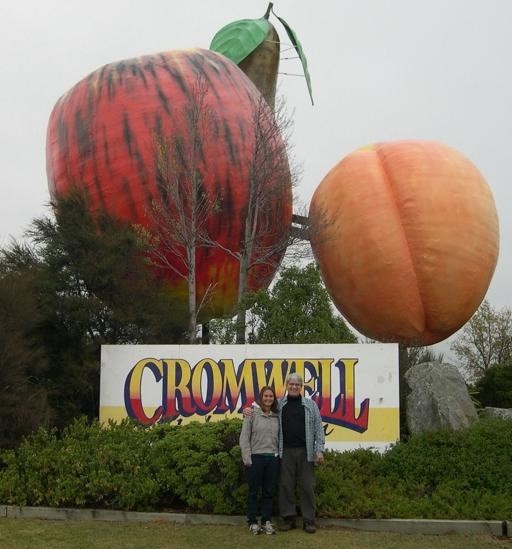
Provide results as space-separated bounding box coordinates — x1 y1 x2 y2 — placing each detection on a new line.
260 521 277 535
279 520 296 531
248 524 261 535
303 520 316 533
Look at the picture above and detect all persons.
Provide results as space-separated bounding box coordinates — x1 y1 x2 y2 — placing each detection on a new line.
240 372 325 535
